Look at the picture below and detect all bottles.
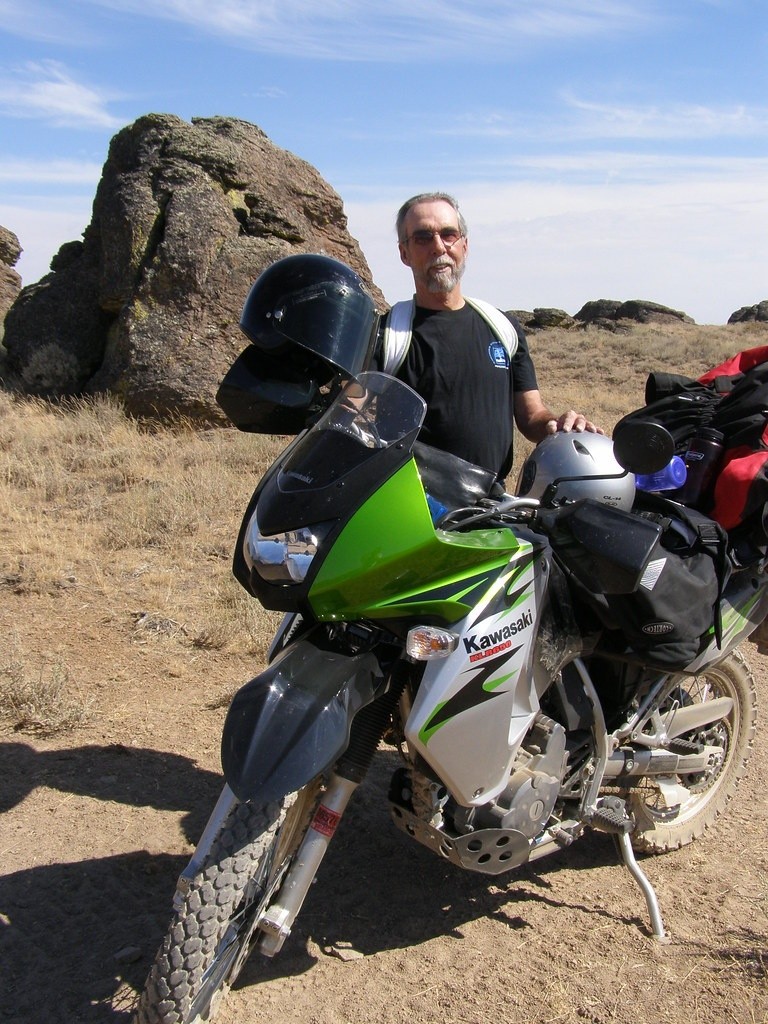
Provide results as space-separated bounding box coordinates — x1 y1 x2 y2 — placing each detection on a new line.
672 426 724 509
635 456 687 491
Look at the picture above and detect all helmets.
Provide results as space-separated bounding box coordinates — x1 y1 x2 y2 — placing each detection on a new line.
239 253 377 382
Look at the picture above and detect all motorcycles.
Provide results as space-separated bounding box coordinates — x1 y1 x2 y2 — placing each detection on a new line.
128 365 767 1024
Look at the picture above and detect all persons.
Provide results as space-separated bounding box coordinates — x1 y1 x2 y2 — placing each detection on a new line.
340 192 605 496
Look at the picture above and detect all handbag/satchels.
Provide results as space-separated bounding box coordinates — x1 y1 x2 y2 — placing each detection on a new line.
605 489 733 671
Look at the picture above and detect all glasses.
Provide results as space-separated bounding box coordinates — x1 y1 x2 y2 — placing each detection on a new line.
408 229 466 249
